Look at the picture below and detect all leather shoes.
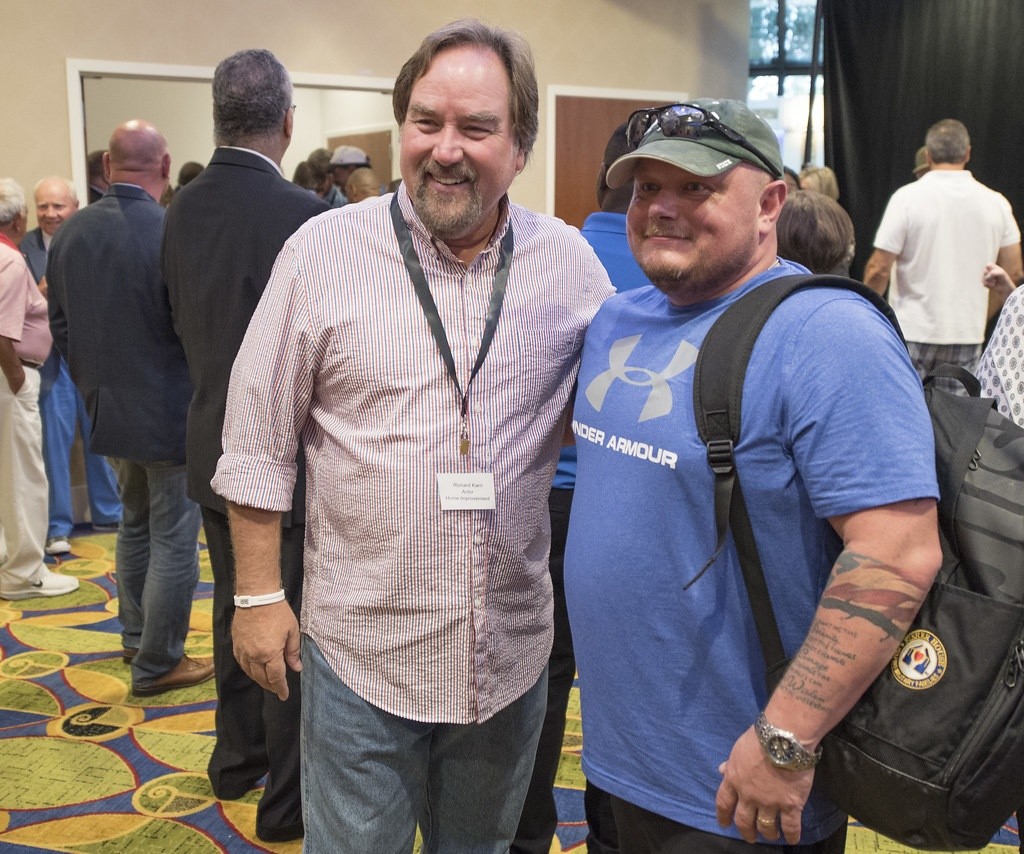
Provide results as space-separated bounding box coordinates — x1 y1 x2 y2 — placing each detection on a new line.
132 653 215 697
122 649 137 664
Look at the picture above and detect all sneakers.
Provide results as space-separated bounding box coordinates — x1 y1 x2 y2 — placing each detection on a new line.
45 536 75 554
90 520 117 531
0 569 80 599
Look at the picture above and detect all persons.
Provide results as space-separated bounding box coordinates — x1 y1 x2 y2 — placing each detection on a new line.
16 175 125 555
45 118 214 697
862 119 1024 396
87 144 388 208
0 176 80 601
776 119 1024 428
159 48 335 842
561 97 943 854
208 18 617 854
510 121 656 854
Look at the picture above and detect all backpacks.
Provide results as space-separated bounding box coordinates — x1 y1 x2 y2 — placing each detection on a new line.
692 264 1023 853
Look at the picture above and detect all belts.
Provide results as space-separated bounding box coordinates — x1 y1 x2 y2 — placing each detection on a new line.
19 359 43 370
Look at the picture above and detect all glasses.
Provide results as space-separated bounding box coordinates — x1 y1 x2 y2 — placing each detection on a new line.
287 103 296 110
627 104 780 176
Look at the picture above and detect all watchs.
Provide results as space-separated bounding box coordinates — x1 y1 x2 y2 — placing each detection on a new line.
754 708 823 772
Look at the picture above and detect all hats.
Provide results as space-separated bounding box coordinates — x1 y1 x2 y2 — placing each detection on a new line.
328 145 370 168
604 95 782 188
914 146 931 173
